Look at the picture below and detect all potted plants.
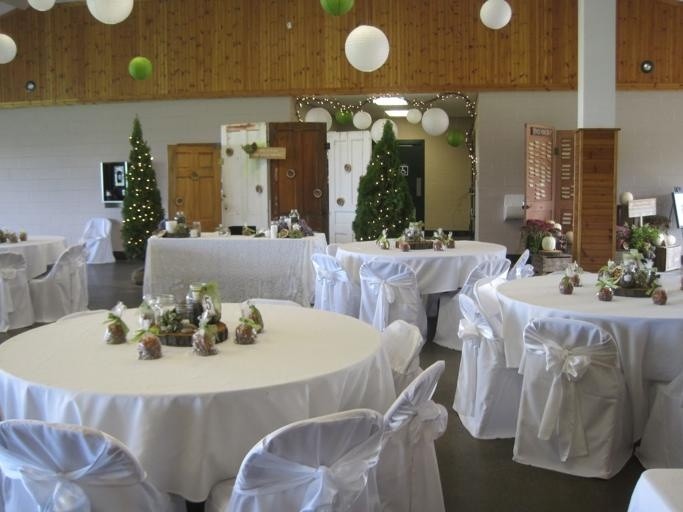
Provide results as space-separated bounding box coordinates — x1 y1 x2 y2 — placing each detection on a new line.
618 223 658 264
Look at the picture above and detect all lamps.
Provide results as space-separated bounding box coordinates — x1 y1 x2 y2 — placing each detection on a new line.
303 106 466 148
129 56 152 81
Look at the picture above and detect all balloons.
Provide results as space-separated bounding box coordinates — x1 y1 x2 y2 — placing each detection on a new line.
302 106 465 148
126 56 153 81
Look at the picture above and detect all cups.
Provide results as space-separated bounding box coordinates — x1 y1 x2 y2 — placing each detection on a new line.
165 220 177 234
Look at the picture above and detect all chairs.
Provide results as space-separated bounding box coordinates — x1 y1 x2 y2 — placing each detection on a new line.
452 292 520 438
383 319 424 397
474 277 507 338
206 407 383 512
512 317 635 478
1 417 164 512
383 361 446 510
310 238 530 347
82 218 116 265
0 234 89 331
638 372 682 469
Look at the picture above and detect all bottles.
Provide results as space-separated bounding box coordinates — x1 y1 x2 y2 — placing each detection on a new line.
267 208 304 240
142 281 207 335
174 210 201 238
217 222 230 238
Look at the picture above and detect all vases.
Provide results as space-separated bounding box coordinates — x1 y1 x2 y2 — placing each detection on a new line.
541 236 557 251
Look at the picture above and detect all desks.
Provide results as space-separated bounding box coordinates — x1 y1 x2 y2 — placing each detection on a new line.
144 225 326 307
